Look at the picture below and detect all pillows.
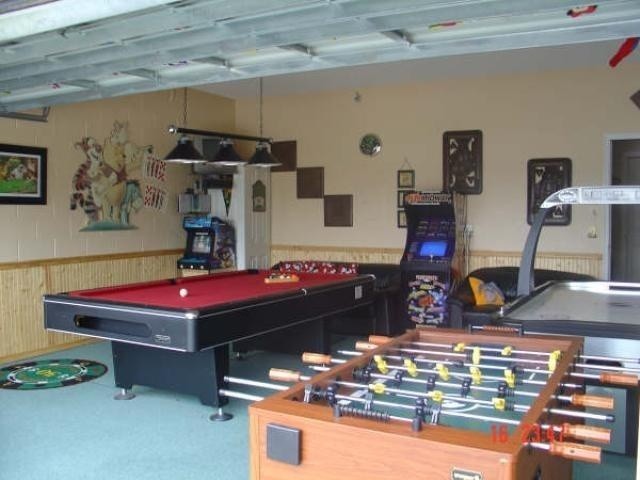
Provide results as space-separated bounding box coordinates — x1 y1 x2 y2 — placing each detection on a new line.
280 261 359 275
488 275 510 297
469 276 504 308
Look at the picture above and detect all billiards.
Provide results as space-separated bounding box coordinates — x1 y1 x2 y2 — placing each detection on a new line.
180 289 188 297
269 272 293 279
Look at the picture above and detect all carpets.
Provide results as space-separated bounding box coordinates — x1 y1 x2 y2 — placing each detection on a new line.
1 359 107 390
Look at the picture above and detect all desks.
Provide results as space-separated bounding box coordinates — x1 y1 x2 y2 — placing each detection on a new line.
41 268 378 424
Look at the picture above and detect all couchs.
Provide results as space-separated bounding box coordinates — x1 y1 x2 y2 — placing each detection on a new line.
273 261 402 336
447 268 599 329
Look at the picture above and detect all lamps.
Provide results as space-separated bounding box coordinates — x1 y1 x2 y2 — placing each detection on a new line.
162 78 282 168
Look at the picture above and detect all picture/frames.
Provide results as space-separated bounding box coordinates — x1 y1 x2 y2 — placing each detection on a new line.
397 169 415 228
527 157 571 225
0 105 51 122
440 129 484 195
0 143 47 205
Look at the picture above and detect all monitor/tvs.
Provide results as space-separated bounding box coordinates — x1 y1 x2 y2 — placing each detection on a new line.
420 240 448 257
191 234 211 254
